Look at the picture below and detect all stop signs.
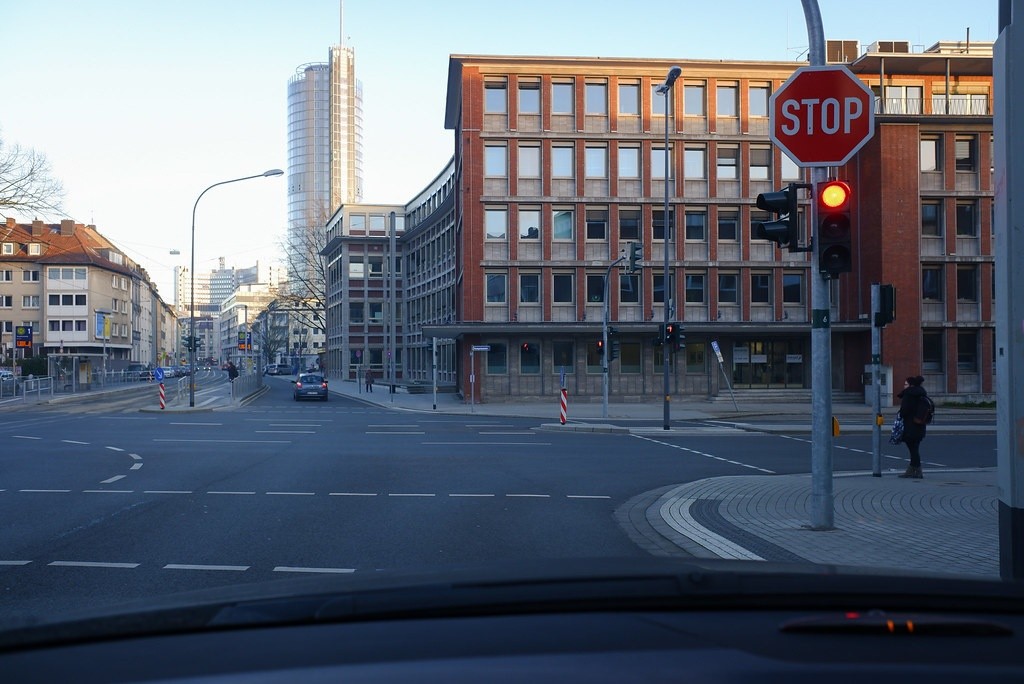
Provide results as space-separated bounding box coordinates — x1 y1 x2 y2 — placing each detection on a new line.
768 64 878 168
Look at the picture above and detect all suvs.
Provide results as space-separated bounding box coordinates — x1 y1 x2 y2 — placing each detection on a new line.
123 364 147 381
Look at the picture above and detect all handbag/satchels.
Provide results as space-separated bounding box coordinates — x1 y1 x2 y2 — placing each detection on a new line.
889 412 905 445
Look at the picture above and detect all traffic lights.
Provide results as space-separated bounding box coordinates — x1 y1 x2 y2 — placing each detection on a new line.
609 325 619 363
597 340 604 356
629 242 643 273
815 179 854 279
194 335 201 351
757 181 800 250
184 335 192 350
671 323 685 352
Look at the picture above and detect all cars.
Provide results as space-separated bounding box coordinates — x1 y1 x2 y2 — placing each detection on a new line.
0 370 17 383
291 374 328 402
204 365 212 371
141 364 201 380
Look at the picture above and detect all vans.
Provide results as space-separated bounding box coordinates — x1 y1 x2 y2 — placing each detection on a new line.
268 363 294 375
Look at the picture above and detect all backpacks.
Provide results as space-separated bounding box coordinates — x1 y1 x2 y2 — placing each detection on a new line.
908 390 935 424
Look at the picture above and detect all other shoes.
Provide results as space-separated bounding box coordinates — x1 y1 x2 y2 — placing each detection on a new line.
898 465 915 478
907 466 923 479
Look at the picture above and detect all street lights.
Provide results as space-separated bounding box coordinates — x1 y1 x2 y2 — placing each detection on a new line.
189 169 284 408
236 304 248 371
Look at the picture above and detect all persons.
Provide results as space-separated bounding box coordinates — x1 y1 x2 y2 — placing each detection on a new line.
898 375 932 480
365 367 374 393
228 361 238 394
